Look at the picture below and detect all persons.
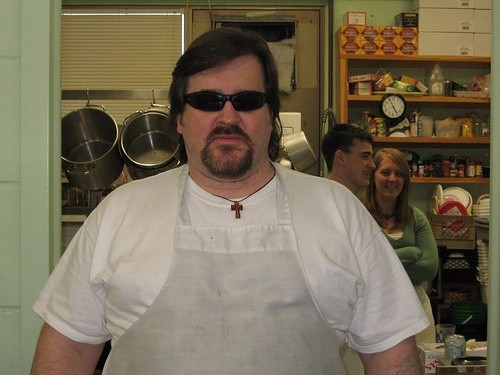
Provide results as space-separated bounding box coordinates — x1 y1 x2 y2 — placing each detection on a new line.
321 123 377 193
29 27 431 375
359 147 439 347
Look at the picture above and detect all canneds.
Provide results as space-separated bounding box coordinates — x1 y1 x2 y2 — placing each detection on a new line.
444 335 466 365
475 161 483 177
445 81 452 95
457 157 466 178
467 158 476 177
442 159 450 177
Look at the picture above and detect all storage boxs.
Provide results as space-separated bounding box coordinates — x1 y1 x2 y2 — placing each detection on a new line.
429 212 489 342
417 343 487 374
435 121 462 138
336 0 491 57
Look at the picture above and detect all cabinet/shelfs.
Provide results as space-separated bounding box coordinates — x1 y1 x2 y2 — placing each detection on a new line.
339 56 492 182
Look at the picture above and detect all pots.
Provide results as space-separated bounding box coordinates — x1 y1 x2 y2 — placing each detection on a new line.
61 105 124 191
120 104 180 181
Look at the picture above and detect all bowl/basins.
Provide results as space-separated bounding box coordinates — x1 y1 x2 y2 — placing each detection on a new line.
442 254 470 269
435 121 461 137
281 131 317 171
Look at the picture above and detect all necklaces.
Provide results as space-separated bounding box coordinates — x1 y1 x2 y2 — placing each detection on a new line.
213 173 277 219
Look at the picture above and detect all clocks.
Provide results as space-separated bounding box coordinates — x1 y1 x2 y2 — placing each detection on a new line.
380 93 407 126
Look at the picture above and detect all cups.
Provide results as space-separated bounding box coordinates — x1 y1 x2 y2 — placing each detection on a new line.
436 324 455 343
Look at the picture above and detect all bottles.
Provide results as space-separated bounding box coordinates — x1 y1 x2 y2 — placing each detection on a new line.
409 153 490 178
370 120 377 136
360 111 369 131
429 64 445 95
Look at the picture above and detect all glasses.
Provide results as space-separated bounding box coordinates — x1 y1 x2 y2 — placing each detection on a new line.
183 90 269 112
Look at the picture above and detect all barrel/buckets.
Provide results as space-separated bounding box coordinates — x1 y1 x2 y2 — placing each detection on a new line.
448 301 485 338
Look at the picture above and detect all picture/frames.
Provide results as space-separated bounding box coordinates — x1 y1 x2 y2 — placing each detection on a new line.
347 12 366 26
